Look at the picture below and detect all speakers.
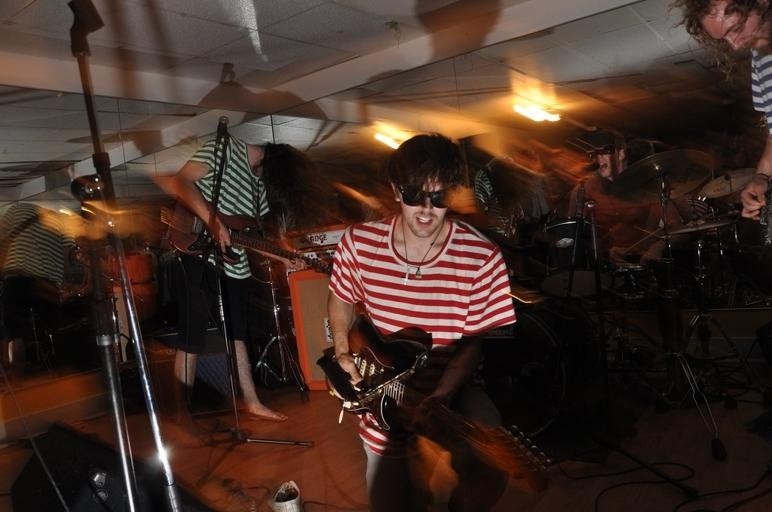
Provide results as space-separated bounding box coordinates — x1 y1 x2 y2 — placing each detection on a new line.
10 422 221 512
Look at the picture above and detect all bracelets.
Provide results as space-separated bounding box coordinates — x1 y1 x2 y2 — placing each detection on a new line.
753 173 769 179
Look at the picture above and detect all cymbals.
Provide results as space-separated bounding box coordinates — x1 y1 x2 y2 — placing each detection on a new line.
667 217 732 234
702 168 757 198
614 150 716 203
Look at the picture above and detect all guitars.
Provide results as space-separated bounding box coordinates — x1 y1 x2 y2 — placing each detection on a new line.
167 200 331 275
317 314 553 480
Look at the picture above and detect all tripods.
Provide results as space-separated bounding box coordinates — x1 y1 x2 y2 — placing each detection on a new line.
19 308 54 378
559 218 698 499
195 244 315 488
654 231 772 414
614 203 728 460
252 256 311 403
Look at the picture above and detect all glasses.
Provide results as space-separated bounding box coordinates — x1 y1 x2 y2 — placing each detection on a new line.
394 183 458 208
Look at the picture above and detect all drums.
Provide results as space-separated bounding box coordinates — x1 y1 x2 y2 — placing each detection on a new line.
480 293 600 442
541 221 613 297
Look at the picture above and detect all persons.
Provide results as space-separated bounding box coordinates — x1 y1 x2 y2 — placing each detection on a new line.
327 133 517 512
0 201 78 389
160 138 308 449
472 128 712 354
677 0 772 222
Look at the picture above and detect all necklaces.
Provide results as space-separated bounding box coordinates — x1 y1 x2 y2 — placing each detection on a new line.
401 217 444 280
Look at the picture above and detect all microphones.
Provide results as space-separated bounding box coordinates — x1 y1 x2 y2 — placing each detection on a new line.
215 116 229 149
575 162 600 179
